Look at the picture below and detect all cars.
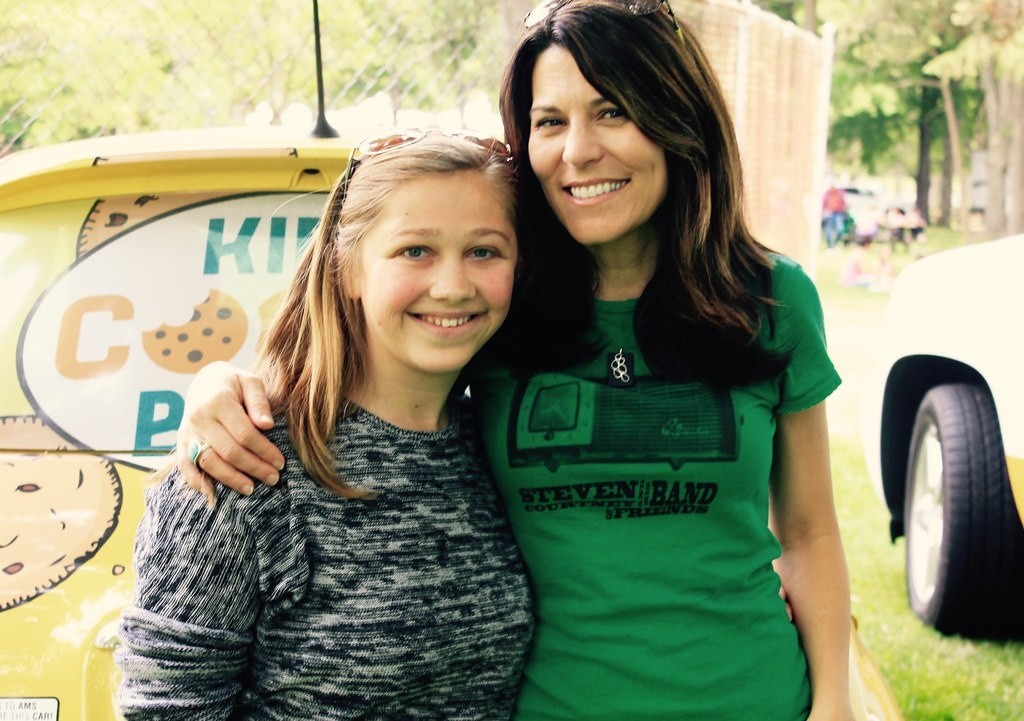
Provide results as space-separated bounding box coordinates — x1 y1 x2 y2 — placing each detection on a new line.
845 229 1024 630
0 0 364 721
821 176 932 250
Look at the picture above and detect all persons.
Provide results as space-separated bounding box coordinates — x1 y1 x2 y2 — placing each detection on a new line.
115 130 795 721
172 0 854 721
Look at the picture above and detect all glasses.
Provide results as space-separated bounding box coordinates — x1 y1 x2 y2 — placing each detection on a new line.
344 128 512 202
524 0 688 49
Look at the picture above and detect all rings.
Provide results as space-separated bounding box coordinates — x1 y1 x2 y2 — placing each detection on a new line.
187 439 211 468
